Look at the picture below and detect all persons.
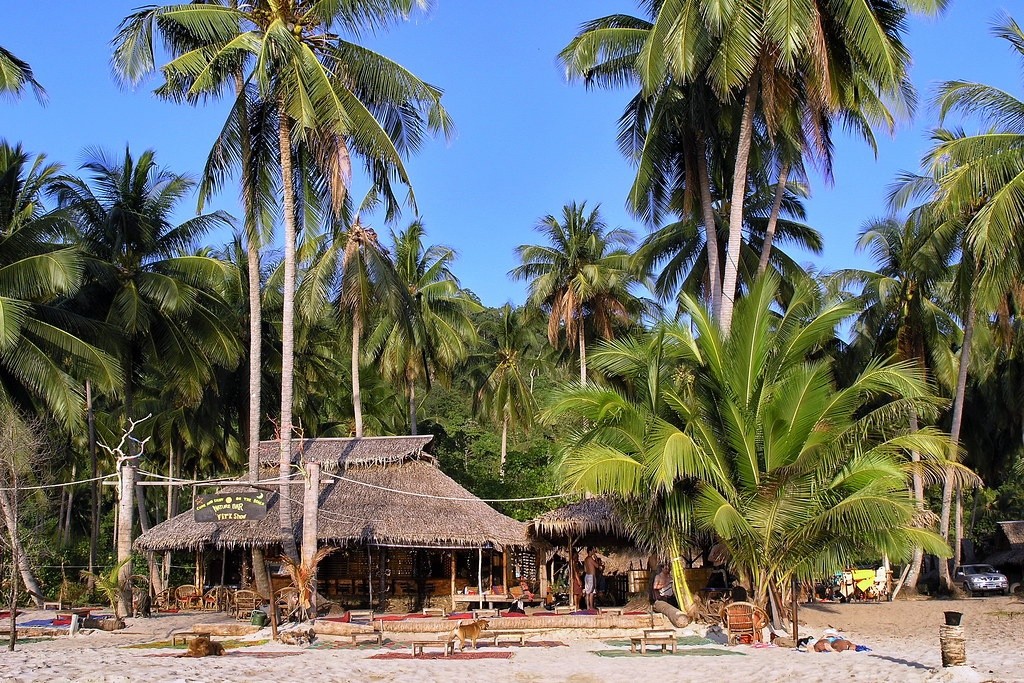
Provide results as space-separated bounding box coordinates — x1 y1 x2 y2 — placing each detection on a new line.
723 585 763 644
569 547 602 611
807 632 856 653
653 564 678 607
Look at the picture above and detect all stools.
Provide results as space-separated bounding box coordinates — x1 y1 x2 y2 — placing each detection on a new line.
596 606 624 615
349 609 374 623
472 608 502 618
630 638 678 655
643 629 677 638
554 606 577 614
375 633 390 639
172 632 210 646
43 602 62 610
493 631 525 645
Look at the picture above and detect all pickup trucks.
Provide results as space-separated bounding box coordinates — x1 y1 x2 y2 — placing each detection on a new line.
951 563 1010 598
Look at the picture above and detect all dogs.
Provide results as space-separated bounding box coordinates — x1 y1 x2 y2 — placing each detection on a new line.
447 619 491 652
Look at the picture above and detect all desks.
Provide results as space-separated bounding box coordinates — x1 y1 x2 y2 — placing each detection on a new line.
423 607 447 617
454 594 545 610
351 631 383 646
411 634 457 656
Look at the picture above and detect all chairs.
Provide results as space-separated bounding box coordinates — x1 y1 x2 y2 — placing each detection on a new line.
274 587 301 623
202 585 229 612
720 602 770 646
175 585 202 611
230 589 268 623
